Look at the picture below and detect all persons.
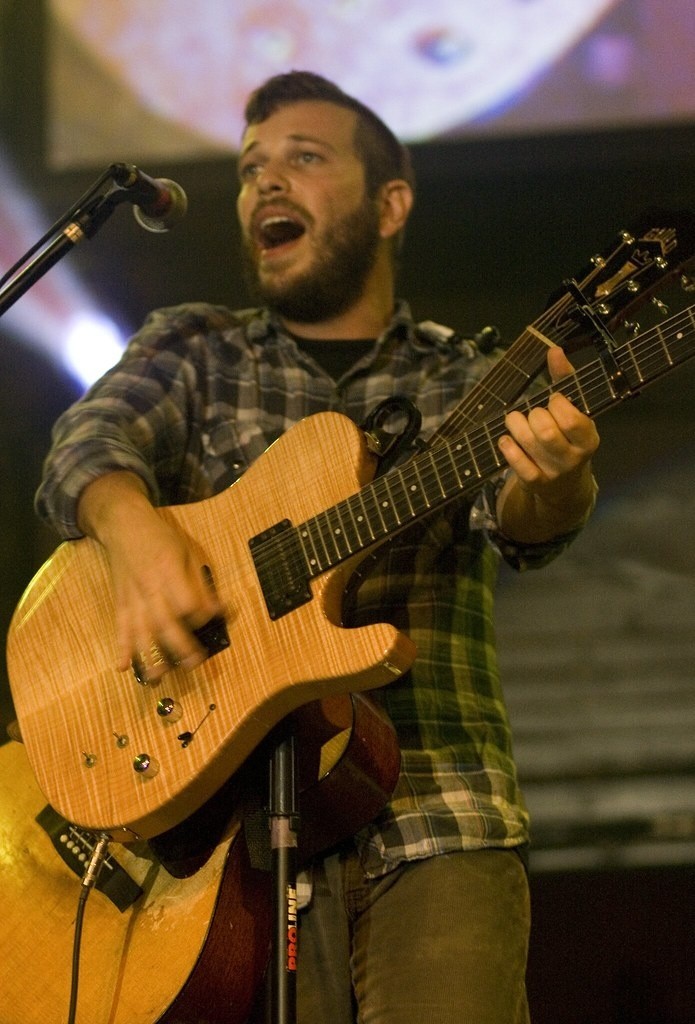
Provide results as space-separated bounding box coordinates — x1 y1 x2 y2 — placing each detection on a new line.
34 72 600 1024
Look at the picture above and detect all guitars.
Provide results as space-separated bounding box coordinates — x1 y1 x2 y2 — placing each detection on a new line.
6 274 695 844
2 222 682 1023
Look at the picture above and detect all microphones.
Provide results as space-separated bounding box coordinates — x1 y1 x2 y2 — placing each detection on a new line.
112 161 187 233
